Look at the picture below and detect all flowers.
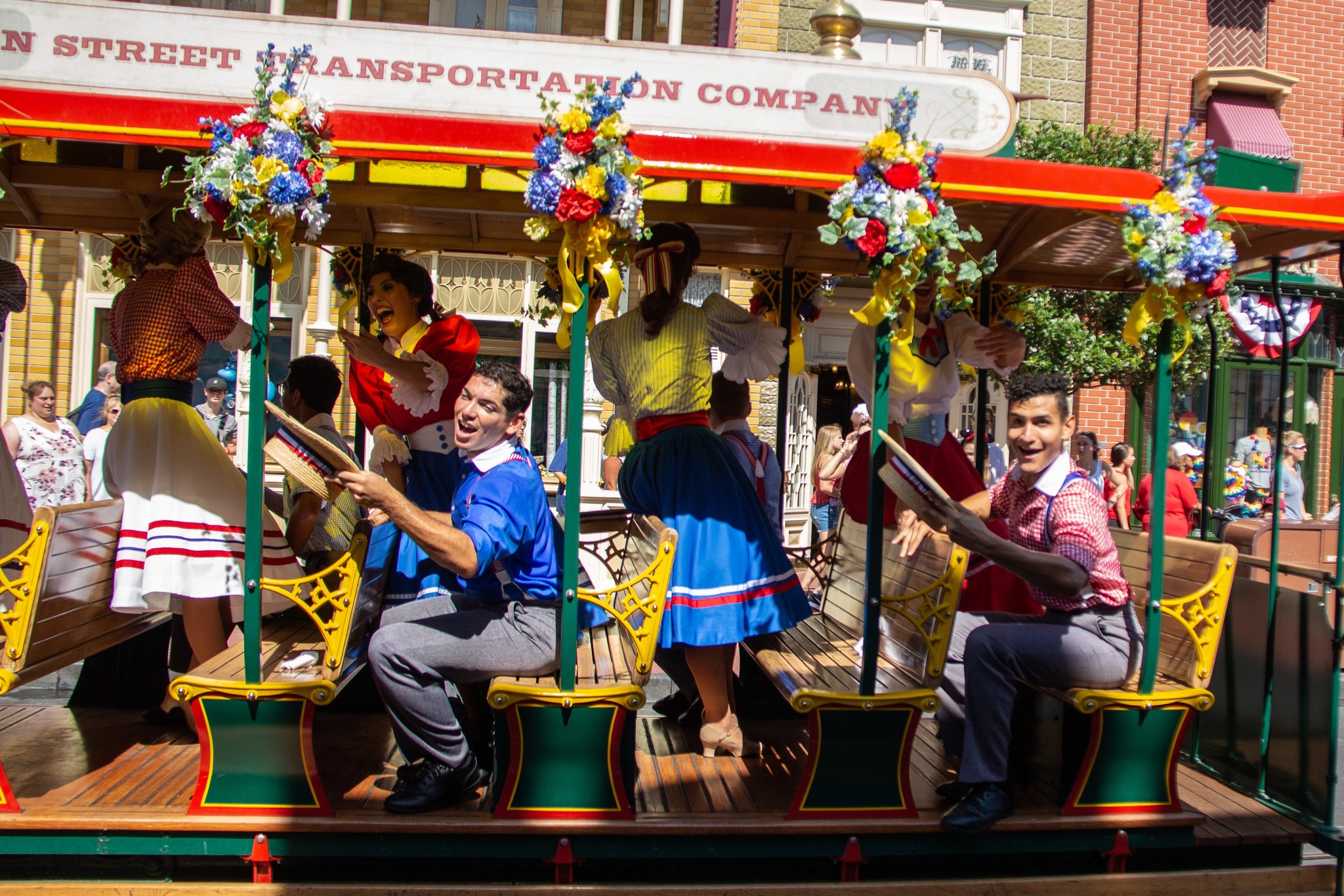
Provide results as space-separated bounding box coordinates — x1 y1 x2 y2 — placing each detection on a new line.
157 39 331 263
816 85 998 323
521 68 652 344
1101 115 1241 300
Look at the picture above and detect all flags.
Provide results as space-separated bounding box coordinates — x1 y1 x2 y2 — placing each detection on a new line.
1215 290 1323 359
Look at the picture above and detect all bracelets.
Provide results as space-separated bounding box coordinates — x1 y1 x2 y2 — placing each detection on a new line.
225 447 230 455
560 476 567 484
838 450 847 456
1196 503 1201 510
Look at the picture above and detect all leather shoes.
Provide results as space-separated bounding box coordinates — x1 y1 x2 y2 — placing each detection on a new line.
385 750 481 814
940 791 1013 833
936 779 971 799
397 754 493 785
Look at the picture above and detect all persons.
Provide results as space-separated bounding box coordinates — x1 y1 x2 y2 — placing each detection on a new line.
102 202 256 737
1074 430 1129 509
798 402 872 612
840 242 1052 626
1224 426 1272 511
1135 440 1214 540
192 377 238 454
83 392 114 502
1103 442 1135 531
77 361 122 444
890 373 1147 829
1 380 87 522
588 220 792 762
322 361 568 809
651 371 786 730
957 404 1008 490
167 351 370 677
1269 431 1315 521
549 413 635 517
337 257 566 776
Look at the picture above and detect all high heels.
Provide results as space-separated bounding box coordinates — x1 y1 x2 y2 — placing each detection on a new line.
160 685 199 735
698 709 766 758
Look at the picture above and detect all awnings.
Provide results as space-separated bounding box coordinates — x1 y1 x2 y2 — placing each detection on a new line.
1207 89 1294 160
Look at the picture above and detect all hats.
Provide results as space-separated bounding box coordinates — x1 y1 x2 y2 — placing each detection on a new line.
1173 442 1202 456
205 377 229 392
262 399 360 504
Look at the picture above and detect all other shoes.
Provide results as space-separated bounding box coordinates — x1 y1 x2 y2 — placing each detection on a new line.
678 678 746 723
652 690 696 716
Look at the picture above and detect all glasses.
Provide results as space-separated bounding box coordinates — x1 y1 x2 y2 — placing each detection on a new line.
276 383 291 397
109 409 119 415
1077 430 1094 437
1293 444 1307 450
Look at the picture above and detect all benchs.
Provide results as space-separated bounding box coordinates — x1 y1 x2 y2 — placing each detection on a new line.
736 509 970 819
1039 525 1238 818
168 511 396 816
486 510 679 820
0 497 174 813
